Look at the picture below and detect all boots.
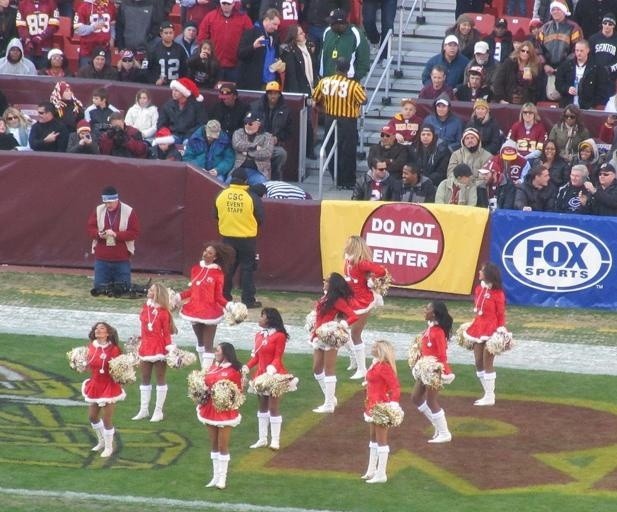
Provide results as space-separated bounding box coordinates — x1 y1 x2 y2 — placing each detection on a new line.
249 411 282 450
149 385 168 422
312 371 337 413
196 343 215 369
345 340 367 379
91 418 115 457
418 400 452 443
361 441 390 483
131 385 152 420
473 371 496 406
205 452 230 489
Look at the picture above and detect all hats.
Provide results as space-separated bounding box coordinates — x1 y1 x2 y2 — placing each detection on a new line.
93 48 133 58
454 164 472 177
381 125 396 135
206 120 221 139
325 10 346 24
580 143 590 149
402 99 489 109
603 13 616 25
151 127 175 146
101 186 119 202
47 48 63 60
243 110 260 122
77 119 91 134
550 0 571 16
220 0 233 4
420 125 433 131
502 147 517 161
170 77 280 102
444 15 506 77
598 163 614 173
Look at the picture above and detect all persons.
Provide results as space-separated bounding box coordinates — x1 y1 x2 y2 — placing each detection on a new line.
352 1 616 217
359 341 400 484
410 299 453 444
84 186 139 290
1 1 397 200
343 234 386 381
173 239 232 371
463 266 509 407
82 321 126 459
214 167 264 309
307 286 358 415
130 283 175 423
244 308 298 449
196 342 243 490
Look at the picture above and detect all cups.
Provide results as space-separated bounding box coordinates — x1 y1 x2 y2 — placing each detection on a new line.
524 68 530 77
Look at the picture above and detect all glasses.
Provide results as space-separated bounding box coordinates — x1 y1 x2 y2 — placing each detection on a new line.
123 58 132 62
565 115 575 119
378 168 387 171
521 50 528 53
39 112 44 114
8 117 16 120
381 134 390 138
598 173 609 176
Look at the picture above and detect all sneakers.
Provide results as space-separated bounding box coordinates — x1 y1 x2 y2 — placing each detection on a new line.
244 301 261 308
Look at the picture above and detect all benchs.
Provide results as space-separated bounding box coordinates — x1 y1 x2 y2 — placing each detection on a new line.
409 0 615 203
2 1 358 180
